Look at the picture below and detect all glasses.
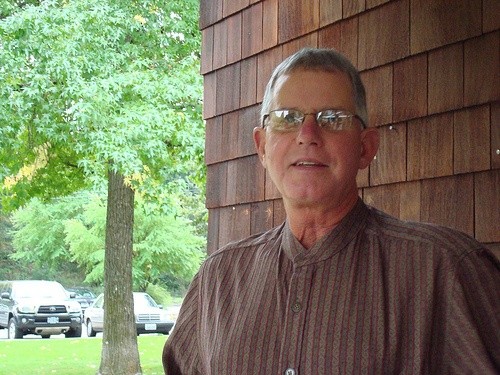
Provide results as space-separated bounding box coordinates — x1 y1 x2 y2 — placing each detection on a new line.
260 108 367 133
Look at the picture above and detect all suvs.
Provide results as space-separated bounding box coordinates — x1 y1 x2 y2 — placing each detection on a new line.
0 279 83 338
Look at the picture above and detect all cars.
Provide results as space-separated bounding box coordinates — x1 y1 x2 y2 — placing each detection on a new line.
65 285 94 324
86 292 175 336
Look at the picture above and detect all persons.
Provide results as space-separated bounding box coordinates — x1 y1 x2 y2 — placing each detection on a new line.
161 47 500 375
